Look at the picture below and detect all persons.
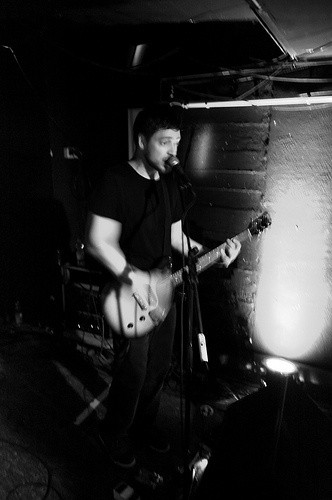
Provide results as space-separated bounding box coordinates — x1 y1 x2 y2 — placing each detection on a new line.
81 106 242 469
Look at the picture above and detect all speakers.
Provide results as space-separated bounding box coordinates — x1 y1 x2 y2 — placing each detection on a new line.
193 376 332 500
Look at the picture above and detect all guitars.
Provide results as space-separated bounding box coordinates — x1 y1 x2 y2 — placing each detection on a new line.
103 211 272 337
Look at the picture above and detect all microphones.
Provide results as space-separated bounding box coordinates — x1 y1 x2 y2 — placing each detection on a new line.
168 156 197 198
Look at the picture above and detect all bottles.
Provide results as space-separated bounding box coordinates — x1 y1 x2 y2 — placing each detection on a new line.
14 301 23 326
75 237 85 266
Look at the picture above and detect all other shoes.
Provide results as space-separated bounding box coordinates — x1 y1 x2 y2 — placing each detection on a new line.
97 430 136 468
136 429 171 453
